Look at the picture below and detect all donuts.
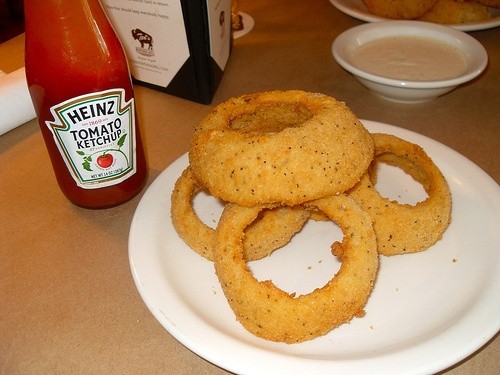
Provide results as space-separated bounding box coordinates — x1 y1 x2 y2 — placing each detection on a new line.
213 193 380 344
171 163 308 262
346 132 452 254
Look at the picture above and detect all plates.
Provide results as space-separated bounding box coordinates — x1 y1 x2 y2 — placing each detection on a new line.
327 0 500 31
126 118 500 375
331 19 489 104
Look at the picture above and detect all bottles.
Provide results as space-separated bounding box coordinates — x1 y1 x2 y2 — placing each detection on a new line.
24 0 147 209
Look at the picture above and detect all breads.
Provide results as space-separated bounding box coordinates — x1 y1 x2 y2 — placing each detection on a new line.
364 0 500 25
188 89 374 207
